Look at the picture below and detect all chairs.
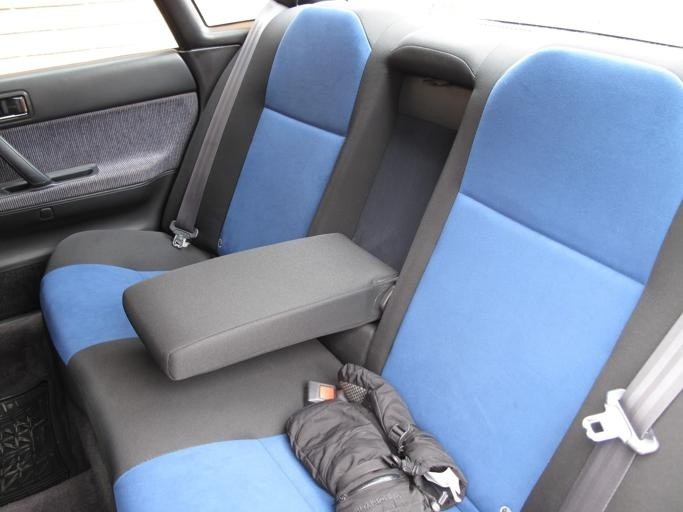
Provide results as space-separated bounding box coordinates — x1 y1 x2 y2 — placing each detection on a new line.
42 1 682 512
